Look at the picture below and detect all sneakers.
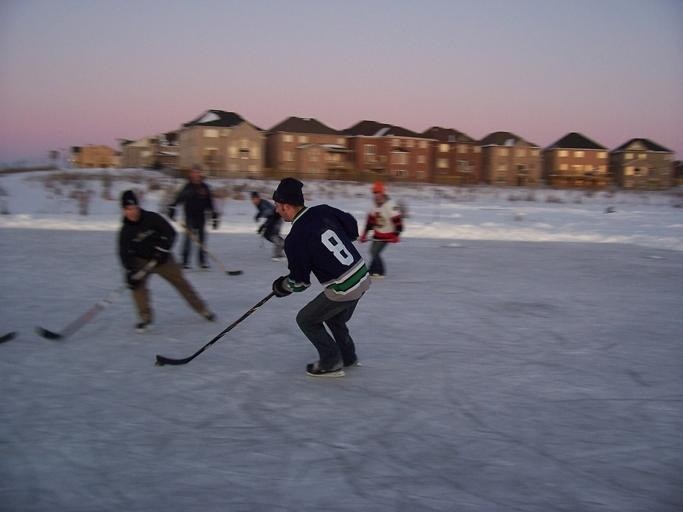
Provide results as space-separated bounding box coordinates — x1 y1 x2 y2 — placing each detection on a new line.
136 321 152 330
368 271 384 278
202 307 216 320
307 360 344 375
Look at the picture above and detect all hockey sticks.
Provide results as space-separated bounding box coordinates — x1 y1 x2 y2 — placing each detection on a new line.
172 217 244 276
156 292 274 365
33 260 156 339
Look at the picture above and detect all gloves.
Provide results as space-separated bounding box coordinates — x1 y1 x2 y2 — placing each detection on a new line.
124 269 140 290
211 218 217 229
360 231 369 243
149 246 168 264
390 234 399 244
272 274 293 297
167 203 176 218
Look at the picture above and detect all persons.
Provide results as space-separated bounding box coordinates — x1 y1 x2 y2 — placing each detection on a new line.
252 192 286 257
117 191 215 329
167 166 220 269
360 180 403 277
272 177 372 378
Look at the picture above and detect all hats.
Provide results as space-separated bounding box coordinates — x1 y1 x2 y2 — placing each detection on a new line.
272 177 305 206
373 180 382 193
121 189 140 206
